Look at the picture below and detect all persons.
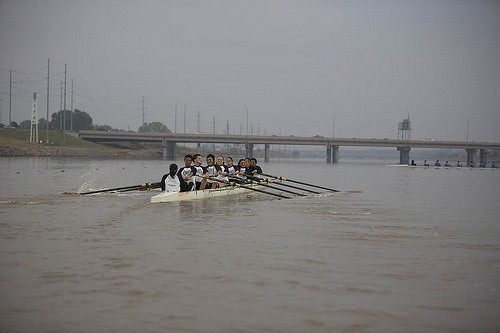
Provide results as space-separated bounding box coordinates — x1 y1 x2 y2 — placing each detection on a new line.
160 164 194 192
225 157 240 184
205 154 222 189
192 154 209 190
177 154 197 191
238 158 262 181
216 156 229 188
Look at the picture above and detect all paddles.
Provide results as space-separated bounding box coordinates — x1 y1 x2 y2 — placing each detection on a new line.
62 181 162 195
197 172 341 199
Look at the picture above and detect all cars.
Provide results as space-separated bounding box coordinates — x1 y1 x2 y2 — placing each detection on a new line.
4 125 16 129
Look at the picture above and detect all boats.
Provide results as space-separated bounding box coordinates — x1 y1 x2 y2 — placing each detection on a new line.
149 177 273 203
